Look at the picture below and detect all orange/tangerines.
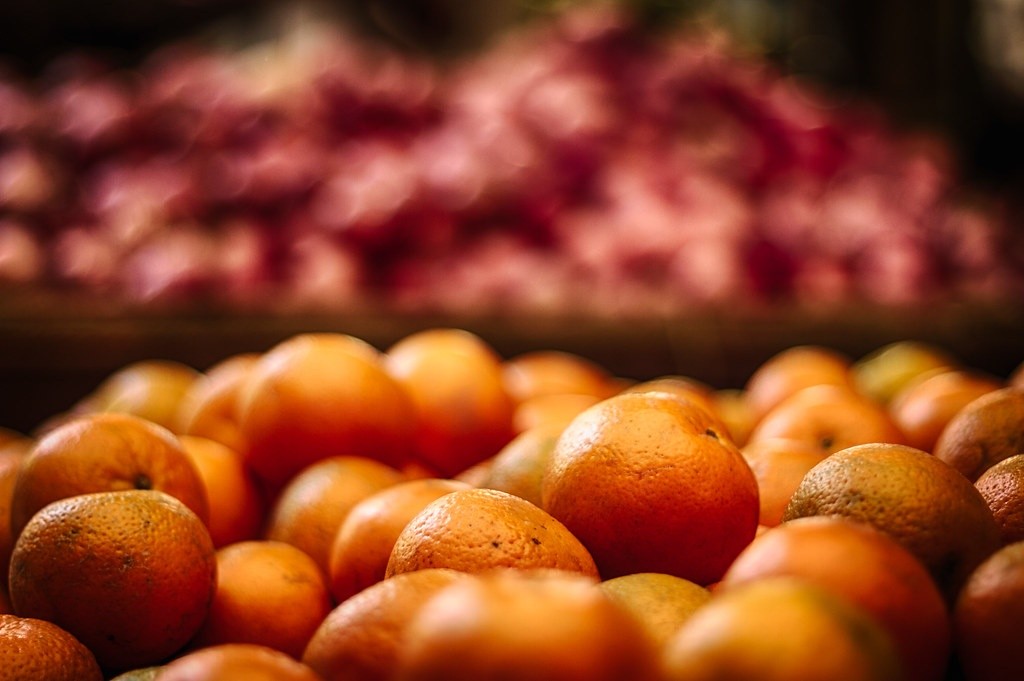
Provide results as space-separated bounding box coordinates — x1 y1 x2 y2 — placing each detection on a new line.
0 329 1024 681
